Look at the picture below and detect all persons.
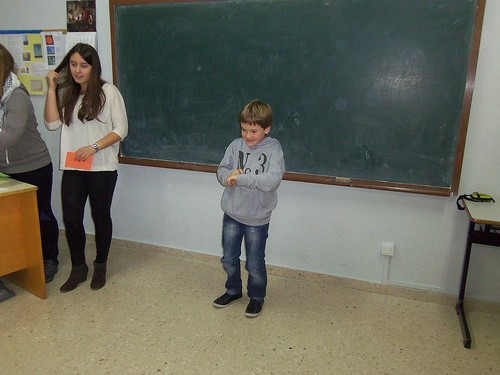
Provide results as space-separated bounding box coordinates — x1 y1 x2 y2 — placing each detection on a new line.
45 43 128 293
0 45 60 284
213 100 286 318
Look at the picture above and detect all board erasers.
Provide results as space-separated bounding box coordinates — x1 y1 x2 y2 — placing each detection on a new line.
334 176 351 185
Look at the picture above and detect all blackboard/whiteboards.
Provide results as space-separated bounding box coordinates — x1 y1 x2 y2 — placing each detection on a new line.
109 1 488 198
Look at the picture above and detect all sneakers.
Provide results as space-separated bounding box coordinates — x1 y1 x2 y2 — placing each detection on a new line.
246 297 264 318
213 292 242 308
44 259 59 282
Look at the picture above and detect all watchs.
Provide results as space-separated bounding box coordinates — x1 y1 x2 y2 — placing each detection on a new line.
91 142 99 153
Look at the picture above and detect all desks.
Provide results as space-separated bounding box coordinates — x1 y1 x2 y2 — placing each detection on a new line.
0 174 47 300
455 199 500 348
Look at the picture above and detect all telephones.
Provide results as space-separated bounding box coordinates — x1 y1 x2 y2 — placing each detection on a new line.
471 192 492 202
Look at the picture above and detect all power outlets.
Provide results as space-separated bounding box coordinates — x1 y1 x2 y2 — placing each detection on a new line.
381 242 394 256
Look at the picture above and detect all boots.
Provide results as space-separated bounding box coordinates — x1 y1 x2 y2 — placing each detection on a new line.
60 263 88 291
91 261 107 289
